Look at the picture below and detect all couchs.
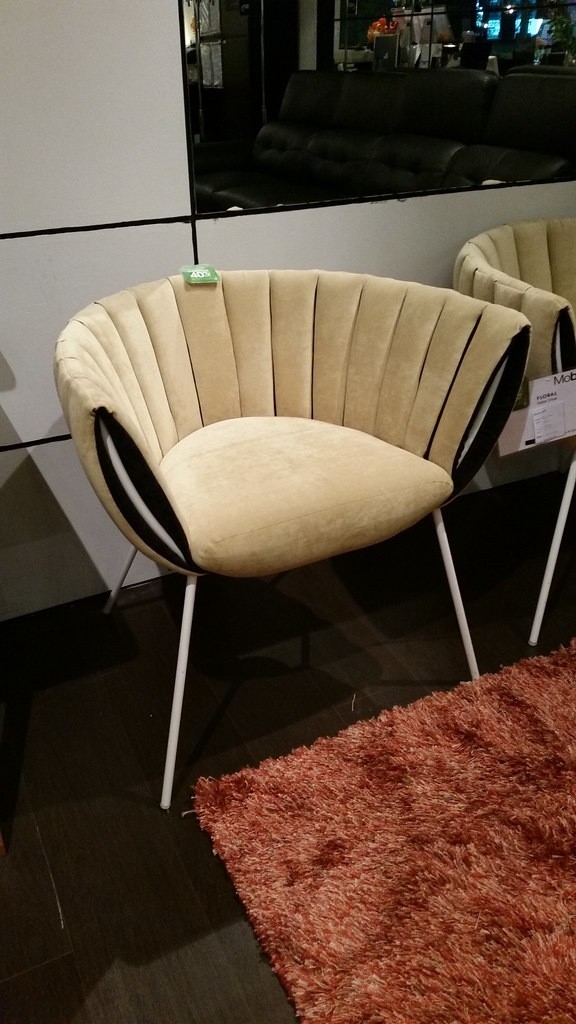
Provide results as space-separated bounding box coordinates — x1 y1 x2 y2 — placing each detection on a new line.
187 64 576 211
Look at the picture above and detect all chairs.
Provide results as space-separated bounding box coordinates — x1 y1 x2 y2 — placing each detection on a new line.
459 217 576 646
52 268 538 814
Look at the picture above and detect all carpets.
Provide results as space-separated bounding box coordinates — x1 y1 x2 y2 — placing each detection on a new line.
191 641 576 1024
